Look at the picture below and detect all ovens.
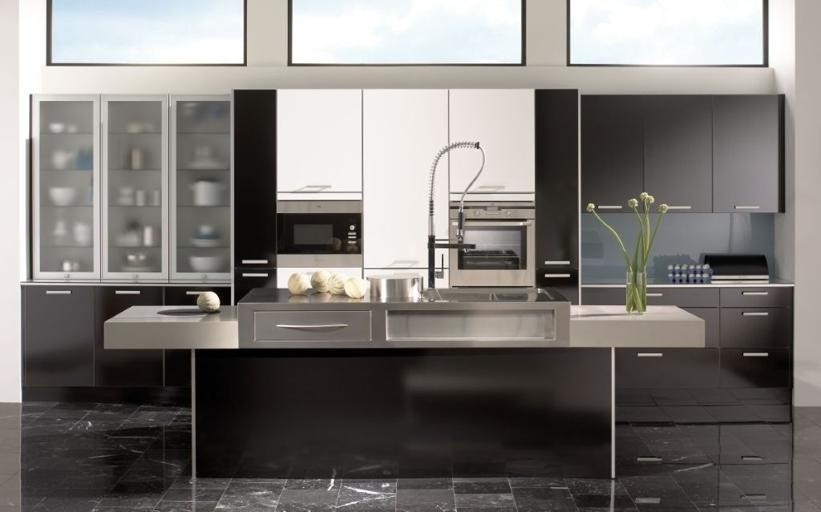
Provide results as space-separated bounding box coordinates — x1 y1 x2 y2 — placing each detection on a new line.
449 202 538 288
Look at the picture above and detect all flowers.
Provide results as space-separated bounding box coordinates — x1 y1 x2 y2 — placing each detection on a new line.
586 191 668 272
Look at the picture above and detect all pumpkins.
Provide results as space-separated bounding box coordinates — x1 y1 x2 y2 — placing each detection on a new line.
311 271 331 292
327 273 347 295
197 291 220 312
288 273 308 294
344 277 366 298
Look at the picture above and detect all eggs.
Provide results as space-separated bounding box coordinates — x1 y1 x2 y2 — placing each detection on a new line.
667 264 710 278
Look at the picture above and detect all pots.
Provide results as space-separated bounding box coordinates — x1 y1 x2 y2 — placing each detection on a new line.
365 273 424 304
187 176 227 208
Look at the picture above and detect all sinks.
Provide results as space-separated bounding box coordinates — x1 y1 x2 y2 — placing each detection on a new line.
435 284 498 302
494 288 556 301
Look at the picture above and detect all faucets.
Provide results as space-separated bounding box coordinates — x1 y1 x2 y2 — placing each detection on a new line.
426 139 485 290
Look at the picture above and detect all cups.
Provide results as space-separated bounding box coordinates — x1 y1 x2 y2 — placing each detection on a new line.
134 189 146 207
143 225 152 247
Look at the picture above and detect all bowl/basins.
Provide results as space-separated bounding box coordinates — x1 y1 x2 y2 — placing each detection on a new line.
47 187 76 207
187 254 224 272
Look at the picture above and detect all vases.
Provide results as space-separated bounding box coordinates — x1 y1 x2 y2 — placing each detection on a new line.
621 267 648 314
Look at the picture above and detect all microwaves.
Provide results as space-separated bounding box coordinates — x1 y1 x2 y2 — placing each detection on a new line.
276 199 364 268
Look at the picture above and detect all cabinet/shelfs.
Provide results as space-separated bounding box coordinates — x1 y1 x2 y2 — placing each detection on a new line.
363 90 450 290
23 94 235 403
579 89 784 214
275 89 363 200
582 282 794 403
449 91 536 202
237 89 580 303
612 422 792 512
